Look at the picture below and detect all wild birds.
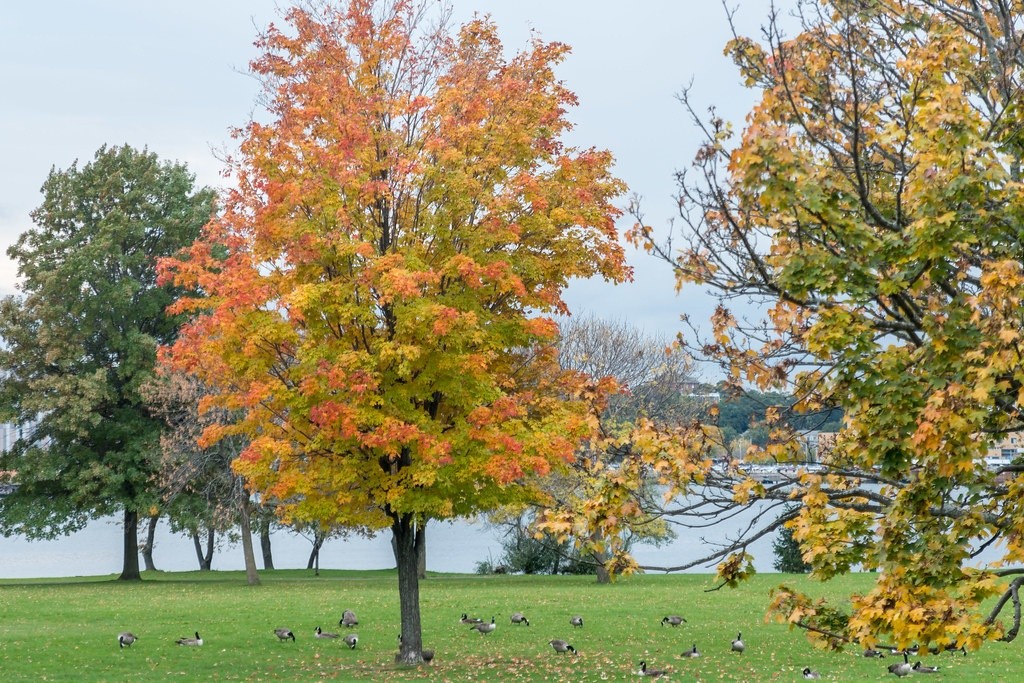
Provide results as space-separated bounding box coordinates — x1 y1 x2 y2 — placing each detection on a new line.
175 630 204 646
117 632 138 648
863 644 920 658
313 626 340 639
888 651 913 678
569 616 584 628
803 667 822 679
470 616 496 635
912 661 942 672
340 609 359 629
661 616 687 627
458 613 484 624
548 639 578 656
342 634 358 649
273 627 295 643
638 661 668 676
680 643 701 657
730 630 746 655
509 613 530 626
